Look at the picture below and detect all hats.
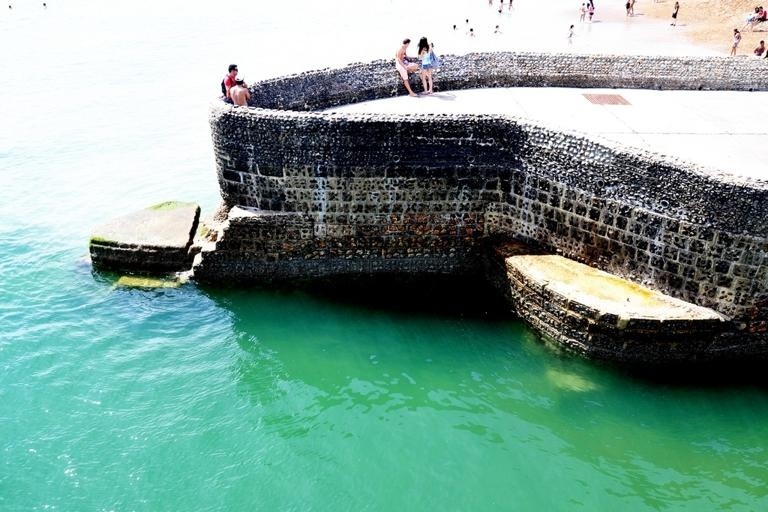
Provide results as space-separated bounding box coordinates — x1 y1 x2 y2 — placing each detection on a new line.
235 75 244 81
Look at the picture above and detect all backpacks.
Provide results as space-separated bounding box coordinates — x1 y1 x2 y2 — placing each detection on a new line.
222 80 226 95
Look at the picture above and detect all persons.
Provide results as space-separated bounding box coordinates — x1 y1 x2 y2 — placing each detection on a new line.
417 36 433 95
579 0 595 23
730 6 768 58
452 0 514 40
568 25 574 41
224 64 239 104
670 1 679 26
395 38 419 97
229 74 250 107
625 0 635 17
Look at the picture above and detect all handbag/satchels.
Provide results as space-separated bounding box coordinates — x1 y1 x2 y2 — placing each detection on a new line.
430 53 439 68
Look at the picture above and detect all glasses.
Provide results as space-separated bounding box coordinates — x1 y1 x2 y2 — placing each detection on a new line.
234 69 238 71
